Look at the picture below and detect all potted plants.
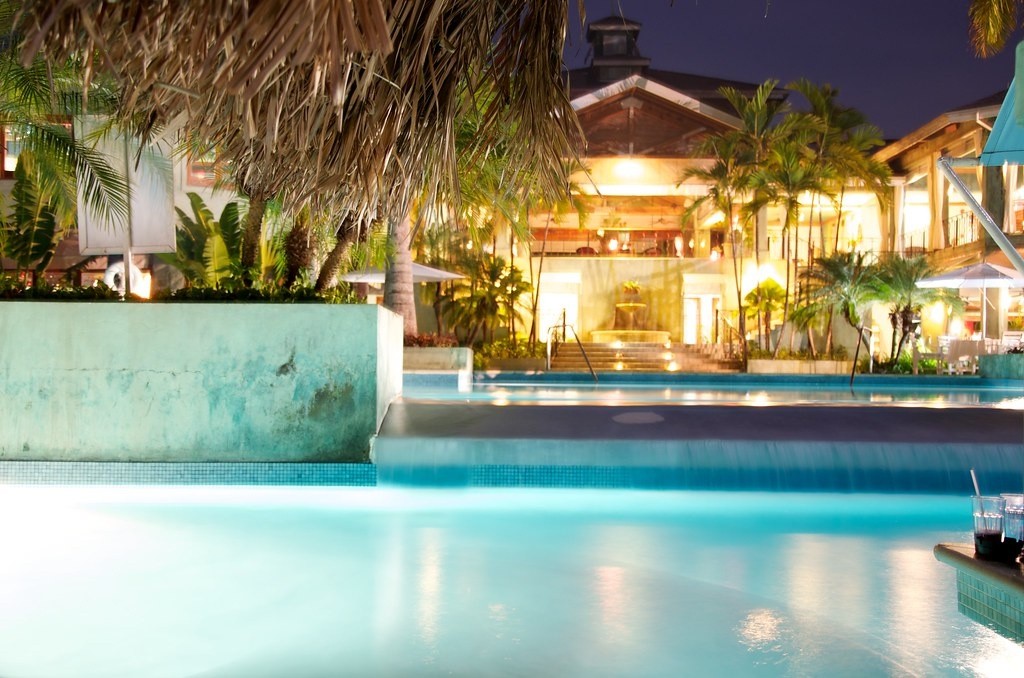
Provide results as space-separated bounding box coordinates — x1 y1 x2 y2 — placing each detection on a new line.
621 280 641 303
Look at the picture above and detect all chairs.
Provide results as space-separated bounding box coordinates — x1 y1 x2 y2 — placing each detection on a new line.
909 332 1024 376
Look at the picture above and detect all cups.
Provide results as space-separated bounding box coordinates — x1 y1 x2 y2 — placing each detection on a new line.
1001 493 1024 556
970 495 1004 556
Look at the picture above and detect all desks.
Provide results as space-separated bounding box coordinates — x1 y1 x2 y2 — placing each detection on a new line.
933 541 1024 648
615 303 646 330
